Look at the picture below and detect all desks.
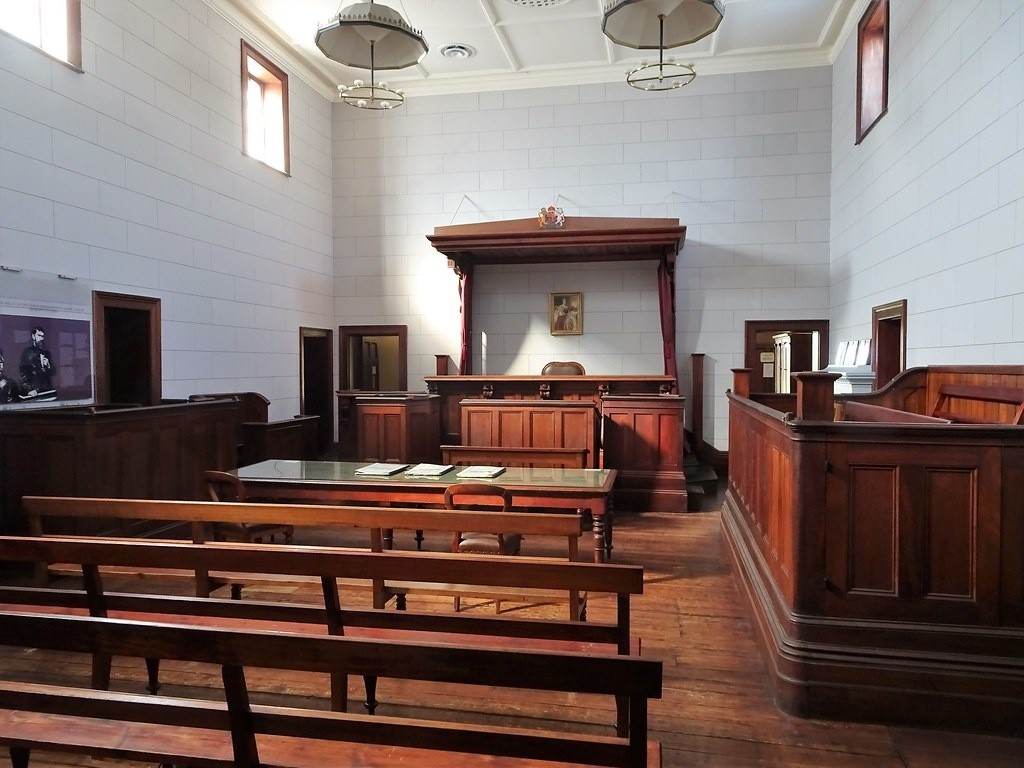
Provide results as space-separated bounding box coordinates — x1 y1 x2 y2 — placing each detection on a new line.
218 459 619 563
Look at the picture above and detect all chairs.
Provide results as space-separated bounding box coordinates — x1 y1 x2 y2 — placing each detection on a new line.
206 470 295 545
932 383 1024 423
443 483 521 615
541 362 585 375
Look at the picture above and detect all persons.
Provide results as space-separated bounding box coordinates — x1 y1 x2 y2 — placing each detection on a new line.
0 355 19 405
17 326 56 401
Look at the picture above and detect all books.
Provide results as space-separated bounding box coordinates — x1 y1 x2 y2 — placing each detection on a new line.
355 461 410 476
19 390 57 403
456 465 506 478
405 462 455 476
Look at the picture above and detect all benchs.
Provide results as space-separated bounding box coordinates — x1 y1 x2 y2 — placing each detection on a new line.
0 496 662 767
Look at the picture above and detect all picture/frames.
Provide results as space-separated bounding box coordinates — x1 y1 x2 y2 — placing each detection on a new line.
549 292 583 335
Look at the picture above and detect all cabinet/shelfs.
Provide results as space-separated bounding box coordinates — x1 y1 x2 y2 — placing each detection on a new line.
771 332 815 392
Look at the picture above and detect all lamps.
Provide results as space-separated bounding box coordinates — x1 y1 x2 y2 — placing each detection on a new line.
313 0 429 110
599 0 725 92
